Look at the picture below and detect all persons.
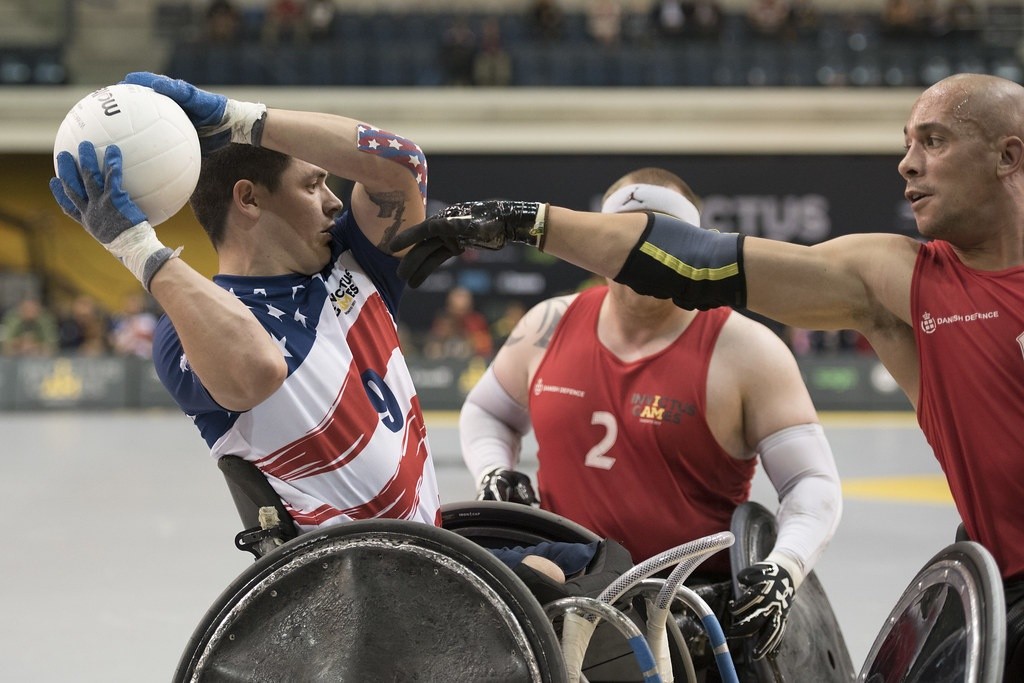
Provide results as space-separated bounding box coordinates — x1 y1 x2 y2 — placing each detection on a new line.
461 168 842 657
396 69 1024 681
49 69 592 607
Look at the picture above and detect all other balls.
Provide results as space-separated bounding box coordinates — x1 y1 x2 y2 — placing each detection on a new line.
53 83 206 227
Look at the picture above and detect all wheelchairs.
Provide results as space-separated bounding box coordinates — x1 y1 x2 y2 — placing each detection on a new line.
173 455 740 681
858 519 1007 681
502 481 859 683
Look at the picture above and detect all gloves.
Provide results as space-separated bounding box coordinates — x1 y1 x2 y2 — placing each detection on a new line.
476 468 536 506
726 561 796 661
50 141 185 295
117 71 267 152
390 200 549 289
